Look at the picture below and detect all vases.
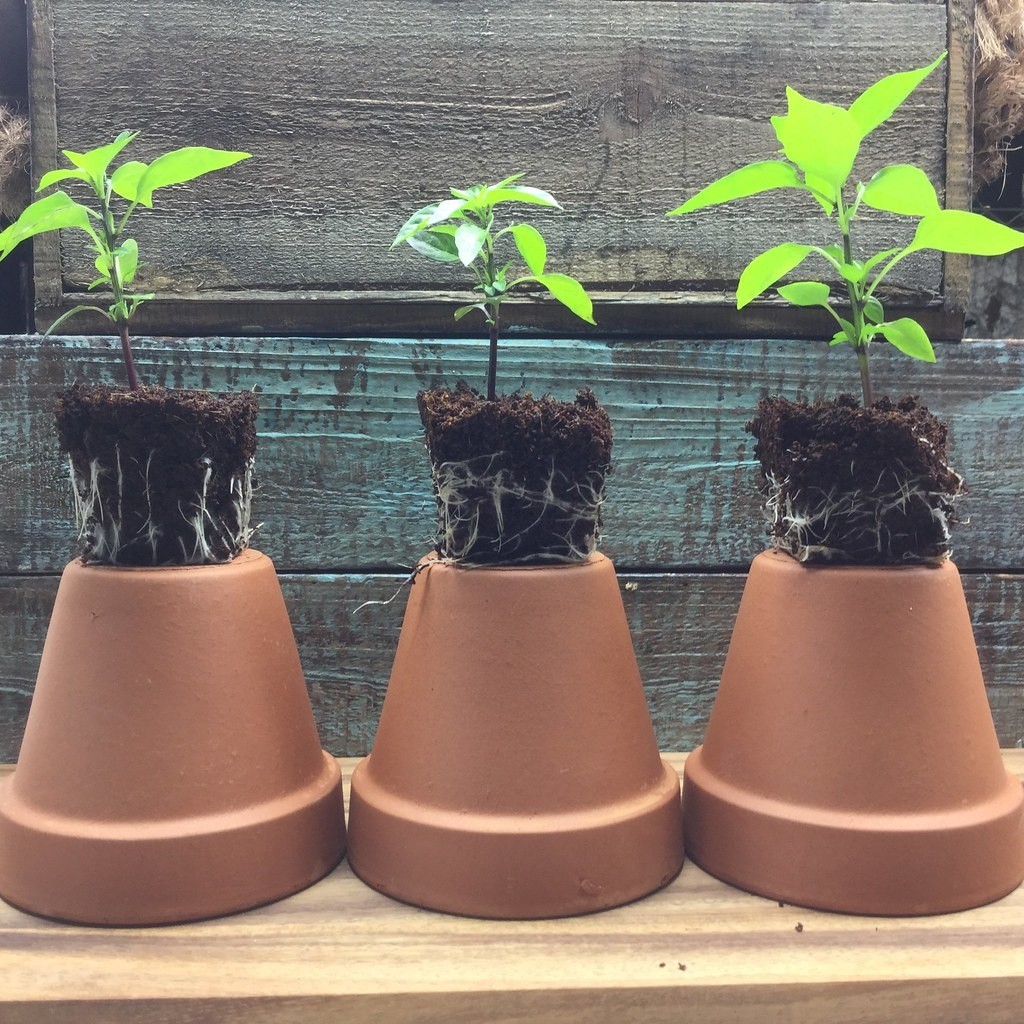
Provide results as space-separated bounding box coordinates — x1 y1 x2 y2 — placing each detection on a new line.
679 548 1023 918
345 548 686 919
0 547 347 927
